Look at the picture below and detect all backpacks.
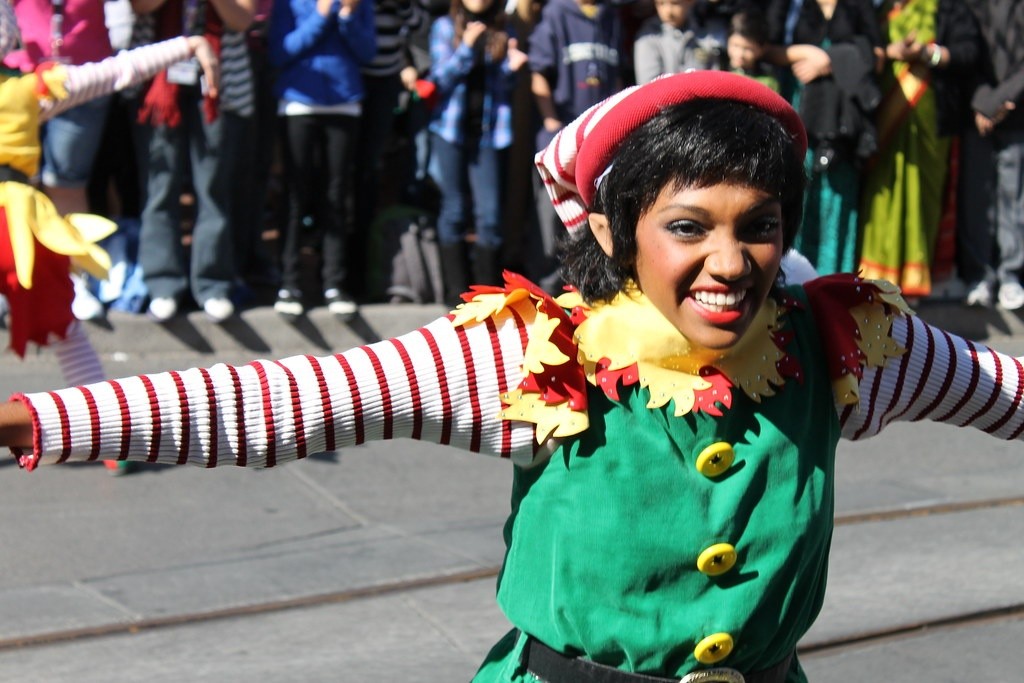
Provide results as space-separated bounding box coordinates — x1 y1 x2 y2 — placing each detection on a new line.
389 206 445 306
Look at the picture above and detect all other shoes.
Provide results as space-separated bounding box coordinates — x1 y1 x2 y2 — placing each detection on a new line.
967 281 992 308
998 281 1024 310
926 277 965 303
149 295 177 322
0 292 6 324
74 274 103 322
331 298 358 316
203 296 235 321
275 301 303 314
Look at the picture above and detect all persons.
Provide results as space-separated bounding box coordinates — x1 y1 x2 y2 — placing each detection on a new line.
0 70 1024 683
0 0 1024 390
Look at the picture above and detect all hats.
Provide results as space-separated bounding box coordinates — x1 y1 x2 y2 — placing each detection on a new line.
0 0 20 69
533 66 807 243
